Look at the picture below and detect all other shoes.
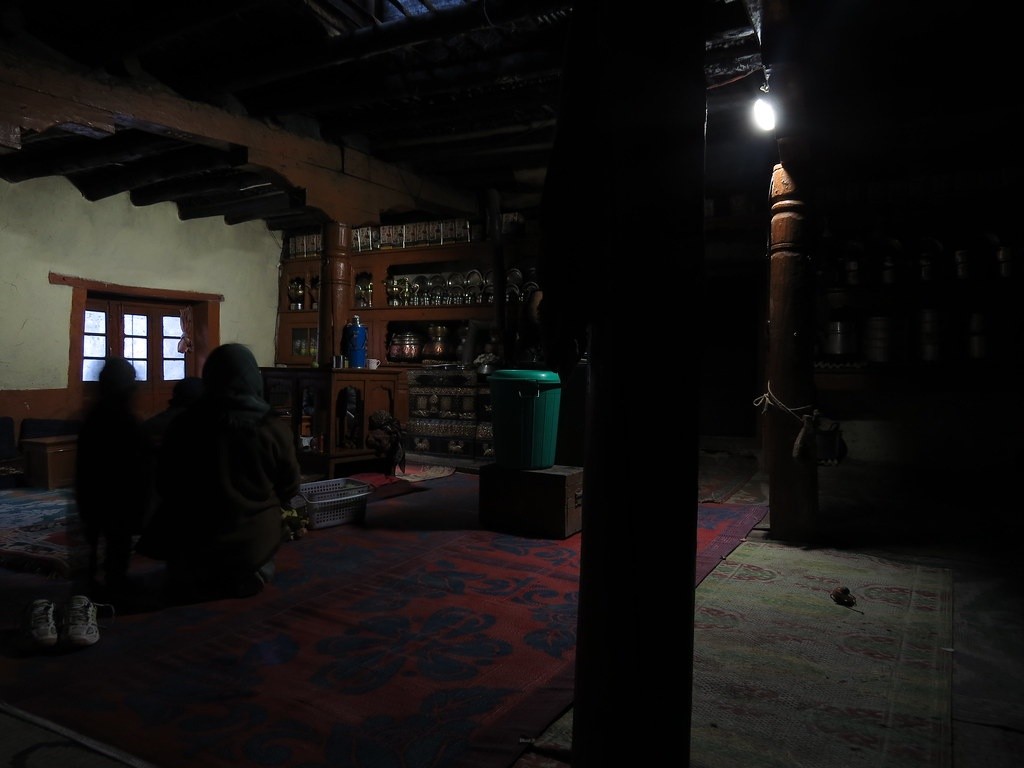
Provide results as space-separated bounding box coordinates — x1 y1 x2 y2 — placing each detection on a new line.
242 571 266 596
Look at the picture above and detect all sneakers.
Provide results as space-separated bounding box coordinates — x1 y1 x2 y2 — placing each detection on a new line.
60 596 116 646
17 598 58 651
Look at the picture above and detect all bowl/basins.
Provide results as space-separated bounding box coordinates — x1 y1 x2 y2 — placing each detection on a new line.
410 269 540 303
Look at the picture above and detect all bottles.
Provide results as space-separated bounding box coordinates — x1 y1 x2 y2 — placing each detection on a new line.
345 315 367 368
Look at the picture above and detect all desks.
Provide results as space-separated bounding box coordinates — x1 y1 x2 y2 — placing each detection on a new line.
19 434 80 490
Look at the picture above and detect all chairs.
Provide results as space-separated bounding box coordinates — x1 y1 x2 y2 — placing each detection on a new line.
0 416 29 491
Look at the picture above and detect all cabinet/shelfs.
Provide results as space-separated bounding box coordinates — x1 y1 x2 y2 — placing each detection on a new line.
257 365 402 480
274 238 524 476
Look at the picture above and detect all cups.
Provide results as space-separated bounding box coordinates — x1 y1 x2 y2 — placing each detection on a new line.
333 355 345 369
366 358 381 371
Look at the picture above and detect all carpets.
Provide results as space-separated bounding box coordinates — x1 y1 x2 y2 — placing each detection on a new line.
538 539 957 768
0 471 431 581
694 448 762 503
0 472 771 768
394 463 458 484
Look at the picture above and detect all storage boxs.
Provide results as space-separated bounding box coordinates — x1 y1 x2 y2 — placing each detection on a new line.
299 478 379 530
478 465 584 539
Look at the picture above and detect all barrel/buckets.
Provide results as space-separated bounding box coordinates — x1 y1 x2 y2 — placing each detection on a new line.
822 242 1011 361
485 369 562 472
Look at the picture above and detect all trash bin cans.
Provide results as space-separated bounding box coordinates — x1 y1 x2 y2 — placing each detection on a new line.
484 367 562 471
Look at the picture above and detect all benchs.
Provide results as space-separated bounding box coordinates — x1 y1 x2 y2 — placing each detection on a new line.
19 415 83 451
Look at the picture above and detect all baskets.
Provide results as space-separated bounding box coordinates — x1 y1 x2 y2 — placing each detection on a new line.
297 477 375 529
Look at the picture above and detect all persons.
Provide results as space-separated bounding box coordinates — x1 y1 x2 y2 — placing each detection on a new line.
72 343 301 606
